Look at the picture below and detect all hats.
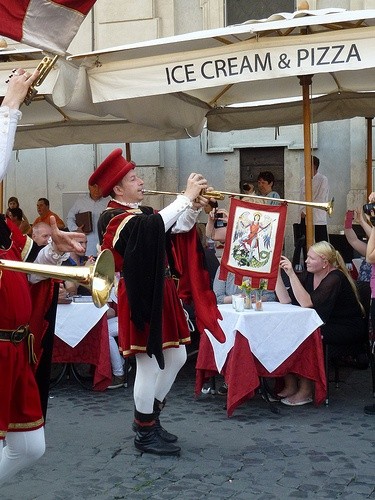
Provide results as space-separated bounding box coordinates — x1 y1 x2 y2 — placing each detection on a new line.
90 148 136 199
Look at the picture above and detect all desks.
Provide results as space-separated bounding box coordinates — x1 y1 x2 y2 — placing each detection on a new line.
48 295 113 393
195 300 327 414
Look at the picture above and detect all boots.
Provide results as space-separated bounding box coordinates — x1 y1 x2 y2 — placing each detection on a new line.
132 396 178 442
133 406 180 457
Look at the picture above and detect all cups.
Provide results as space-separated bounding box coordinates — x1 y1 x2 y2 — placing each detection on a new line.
231 294 245 312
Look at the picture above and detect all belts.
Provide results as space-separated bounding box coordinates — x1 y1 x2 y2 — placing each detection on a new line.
0 324 29 343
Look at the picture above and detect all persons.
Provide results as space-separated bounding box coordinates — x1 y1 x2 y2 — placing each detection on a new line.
206 197 265 242
4 197 32 235
300 156 329 242
32 221 80 299
67 229 95 295
262 242 364 405
212 263 275 394
345 192 375 413
34 198 65 228
89 243 127 388
67 182 111 257
196 226 219 281
87 147 216 455
244 170 279 206
0 67 86 483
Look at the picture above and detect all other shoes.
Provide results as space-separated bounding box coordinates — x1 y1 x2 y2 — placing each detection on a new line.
106 375 128 388
218 382 228 395
281 394 314 407
365 404 375 415
262 392 284 402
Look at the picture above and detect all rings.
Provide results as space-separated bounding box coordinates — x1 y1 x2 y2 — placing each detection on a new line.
14 71 19 75
23 74 28 78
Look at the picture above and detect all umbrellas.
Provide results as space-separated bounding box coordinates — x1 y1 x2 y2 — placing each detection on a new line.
0 36 207 149
64 0 375 252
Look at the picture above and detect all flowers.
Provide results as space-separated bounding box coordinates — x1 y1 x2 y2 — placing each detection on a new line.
240 282 251 295
256 280 268 295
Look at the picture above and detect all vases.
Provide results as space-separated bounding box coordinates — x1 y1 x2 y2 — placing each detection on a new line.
242 287 251 308
255 289 264 311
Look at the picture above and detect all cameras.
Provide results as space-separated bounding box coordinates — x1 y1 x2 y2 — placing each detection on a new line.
214 213 223 218
363 203 375 215
